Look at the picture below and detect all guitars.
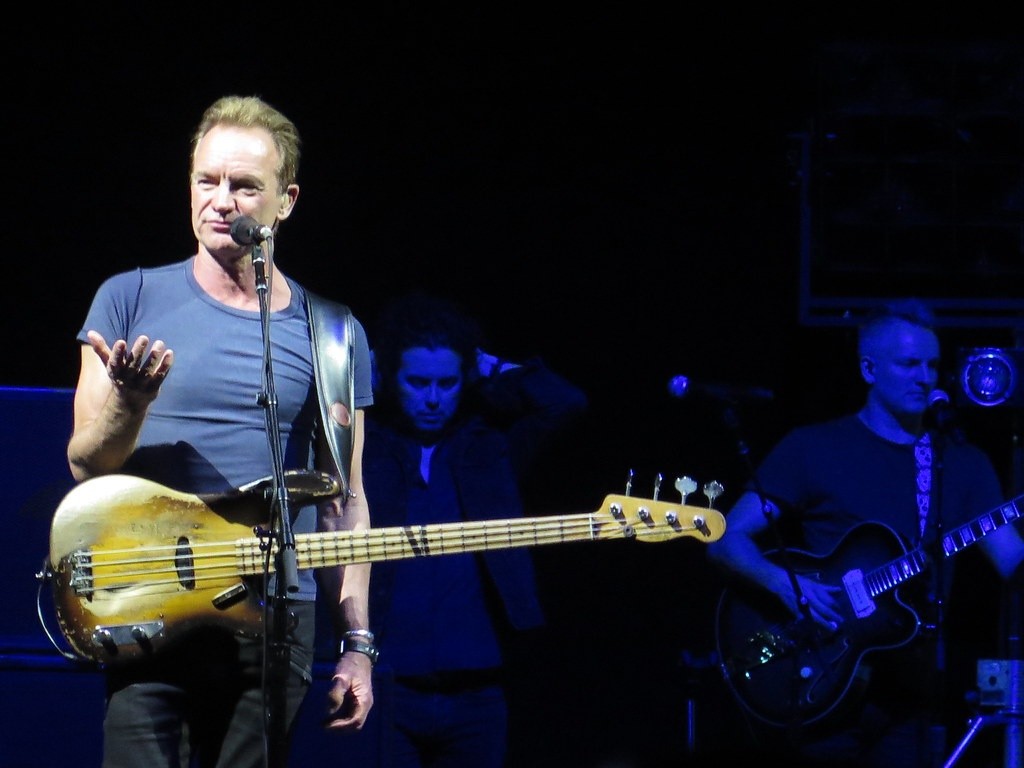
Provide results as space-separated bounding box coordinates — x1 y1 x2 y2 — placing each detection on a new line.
712 492 1024 730
33 467 727 668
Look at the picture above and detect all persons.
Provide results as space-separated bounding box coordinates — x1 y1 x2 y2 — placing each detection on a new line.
362 314 548 768
66 92 375 768
706 297 1024 768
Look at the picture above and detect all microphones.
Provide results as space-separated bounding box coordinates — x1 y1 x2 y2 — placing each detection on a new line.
927 389 949 432
229 215 273 246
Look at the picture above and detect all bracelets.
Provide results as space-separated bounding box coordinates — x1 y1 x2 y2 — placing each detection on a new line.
344 628 375 643
491 356 507 374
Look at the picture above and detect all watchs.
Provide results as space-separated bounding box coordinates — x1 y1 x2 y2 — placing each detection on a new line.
346 643 382 663
